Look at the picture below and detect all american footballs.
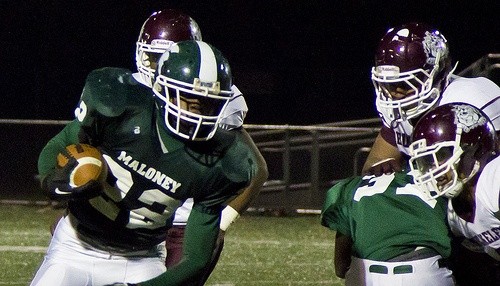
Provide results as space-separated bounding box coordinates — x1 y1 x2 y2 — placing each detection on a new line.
60 144 107 191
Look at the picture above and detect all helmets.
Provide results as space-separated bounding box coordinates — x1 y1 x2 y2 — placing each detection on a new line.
371 21 449 121
152 40 233 141
136 9 202 87
409 102 498 201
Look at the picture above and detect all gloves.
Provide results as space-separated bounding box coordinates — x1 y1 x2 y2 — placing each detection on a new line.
40 158 100 200
367 157 402 178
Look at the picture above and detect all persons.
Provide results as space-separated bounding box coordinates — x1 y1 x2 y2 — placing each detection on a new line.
132 12 269 285
360 21 500 286
36 42 258 286
408 102 500 269
320 167 457 286
